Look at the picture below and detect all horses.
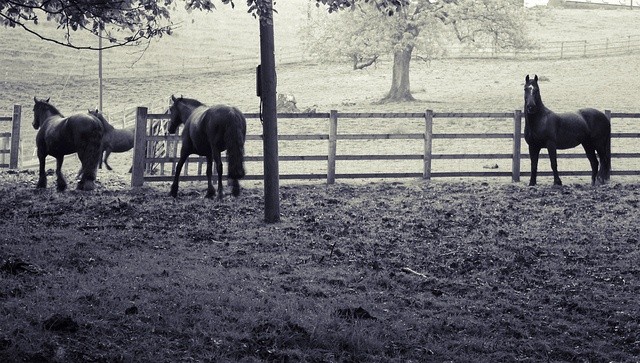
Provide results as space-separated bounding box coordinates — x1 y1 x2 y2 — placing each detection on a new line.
523 72 612 187
168 94 247 200
88 107 148 172
31 96 105 191
